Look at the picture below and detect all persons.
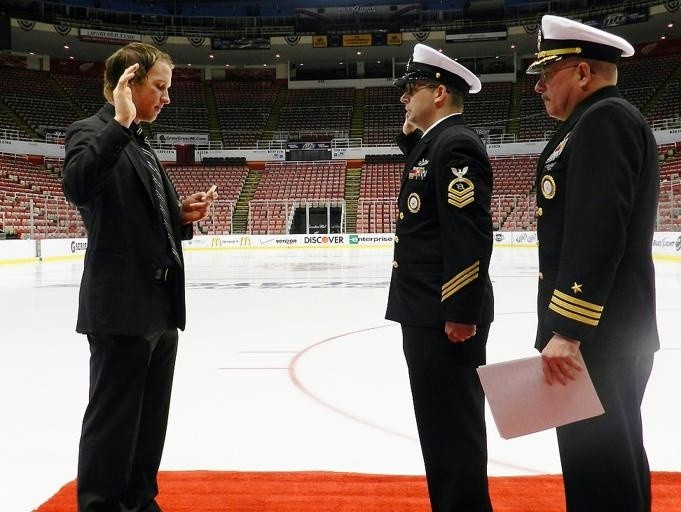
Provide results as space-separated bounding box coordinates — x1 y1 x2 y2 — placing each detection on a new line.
525 14 661 512
385 44 494 512
61 42 219 512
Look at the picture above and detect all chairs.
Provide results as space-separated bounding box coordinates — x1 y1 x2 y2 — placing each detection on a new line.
0 46 681 241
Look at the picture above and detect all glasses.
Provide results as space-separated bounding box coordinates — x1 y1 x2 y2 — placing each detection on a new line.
406 81 449 93
537 64 595 81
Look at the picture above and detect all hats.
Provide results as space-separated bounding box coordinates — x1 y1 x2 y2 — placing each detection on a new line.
392 41 482 93
526 13 635 75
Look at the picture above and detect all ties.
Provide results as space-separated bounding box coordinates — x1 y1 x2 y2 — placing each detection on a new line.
136 125 180 269
